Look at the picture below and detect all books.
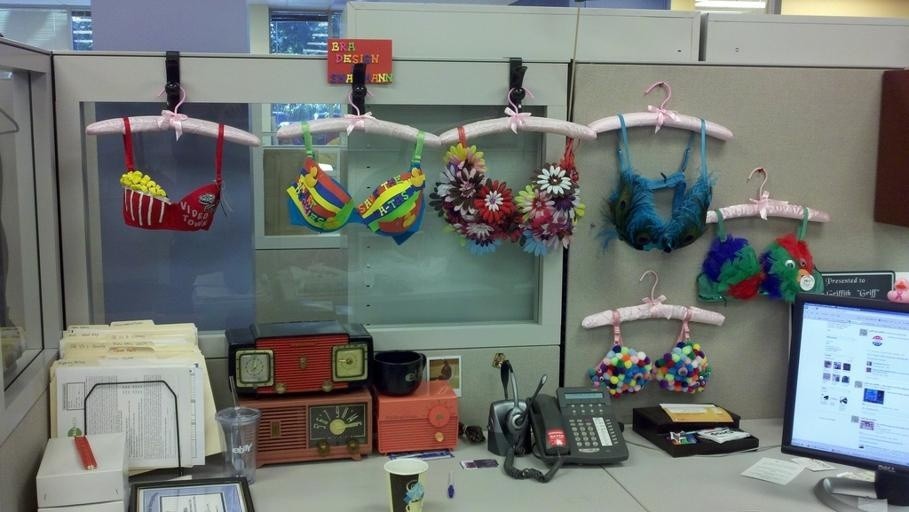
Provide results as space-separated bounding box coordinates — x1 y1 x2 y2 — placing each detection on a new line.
44 318 226 478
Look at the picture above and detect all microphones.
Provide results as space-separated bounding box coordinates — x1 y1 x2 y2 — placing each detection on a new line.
515 373 549 426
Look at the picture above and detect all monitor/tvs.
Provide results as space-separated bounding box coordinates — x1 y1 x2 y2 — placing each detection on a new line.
781 293 909 512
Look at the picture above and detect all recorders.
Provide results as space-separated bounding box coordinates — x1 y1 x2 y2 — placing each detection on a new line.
235 390 373 469
225 319 375 401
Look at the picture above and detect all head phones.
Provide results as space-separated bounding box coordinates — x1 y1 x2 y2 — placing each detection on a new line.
500 359 529 438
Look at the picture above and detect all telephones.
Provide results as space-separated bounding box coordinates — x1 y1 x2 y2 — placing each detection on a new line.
526 386 629 464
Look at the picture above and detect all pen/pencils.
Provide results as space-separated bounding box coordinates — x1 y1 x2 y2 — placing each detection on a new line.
448 472 455 498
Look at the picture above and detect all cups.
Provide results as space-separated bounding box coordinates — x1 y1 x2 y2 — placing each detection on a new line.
216 408 262 486
383 458 428 512
373 350 427 395
